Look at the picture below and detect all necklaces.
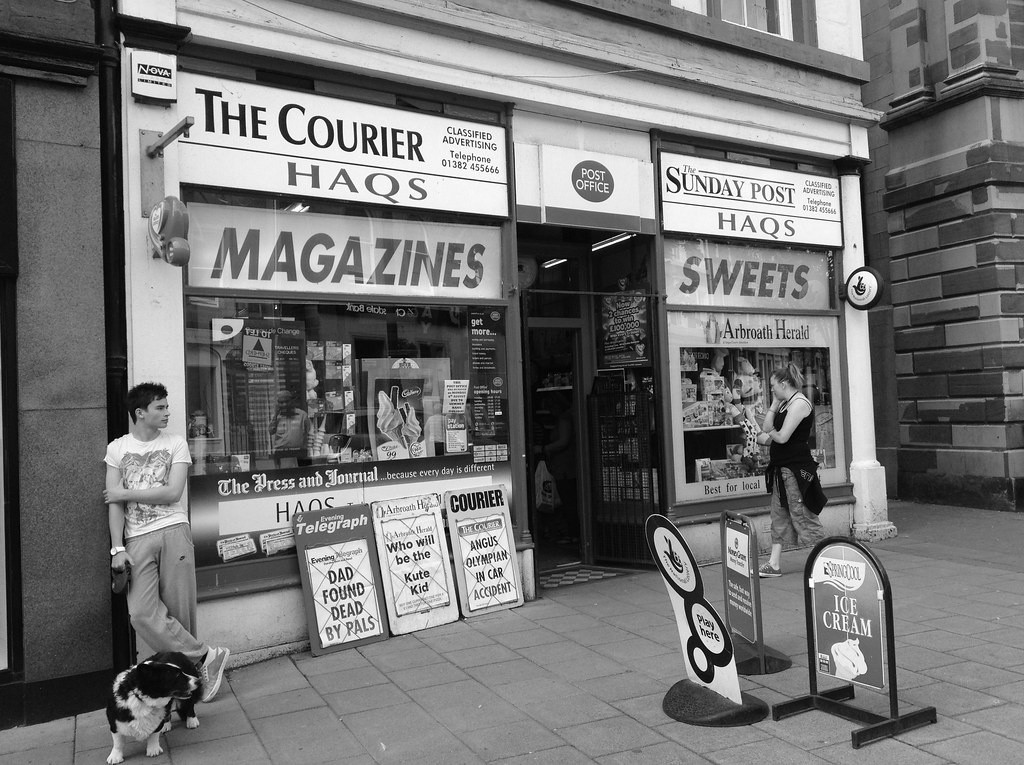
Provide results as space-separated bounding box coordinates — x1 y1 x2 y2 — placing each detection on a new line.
787 390 799 402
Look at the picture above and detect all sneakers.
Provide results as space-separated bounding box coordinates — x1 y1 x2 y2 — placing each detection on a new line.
752 561 784 578
200 645 230 703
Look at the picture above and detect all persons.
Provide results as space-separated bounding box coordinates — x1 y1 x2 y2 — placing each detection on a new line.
758 362 829 577
534 366 580 545
103 383 230 713
268 391 312 467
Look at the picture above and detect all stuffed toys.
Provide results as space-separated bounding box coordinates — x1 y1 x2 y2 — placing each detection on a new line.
711 348 729 376
721 383 758 465
732 357 772 446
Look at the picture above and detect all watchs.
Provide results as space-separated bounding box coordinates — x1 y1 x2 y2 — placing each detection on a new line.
110 547 126 555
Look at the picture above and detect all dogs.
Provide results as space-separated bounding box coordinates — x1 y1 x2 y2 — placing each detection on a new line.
104 650 206 765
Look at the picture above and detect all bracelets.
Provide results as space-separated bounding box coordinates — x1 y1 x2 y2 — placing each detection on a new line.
541 445 545 451
768 409 777 414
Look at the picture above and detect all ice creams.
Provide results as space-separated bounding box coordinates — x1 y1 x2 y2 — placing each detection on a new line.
377 391 407 449
401 407 421 458
831 637 867 680
